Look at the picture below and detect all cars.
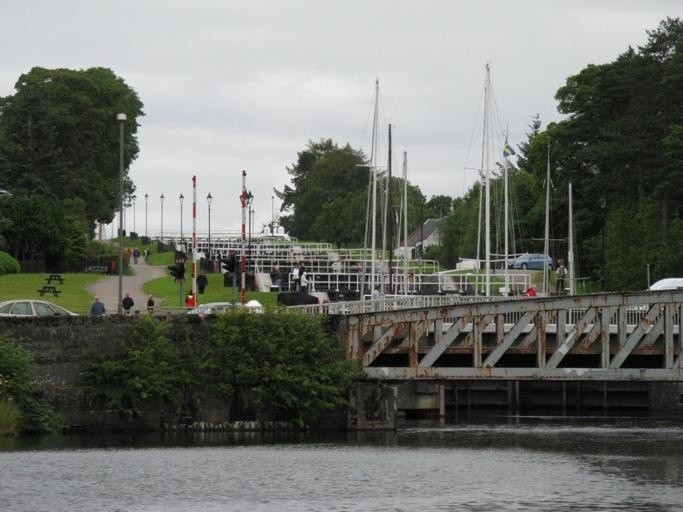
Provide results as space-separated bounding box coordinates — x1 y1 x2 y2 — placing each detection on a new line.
0 299 80 319
629 276 683 311
507 252 554 271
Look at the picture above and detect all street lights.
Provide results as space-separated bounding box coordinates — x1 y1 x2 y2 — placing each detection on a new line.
116 113 127 316
246 190 254 288
159 193 165 244
179 193 185 263
132 195 136 233
145 193 148 243
206 192 213 266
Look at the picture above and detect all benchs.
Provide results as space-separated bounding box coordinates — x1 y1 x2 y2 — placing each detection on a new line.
44 278 64 281
35 290 60 293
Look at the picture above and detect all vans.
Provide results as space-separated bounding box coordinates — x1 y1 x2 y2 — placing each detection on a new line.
186 300 242 318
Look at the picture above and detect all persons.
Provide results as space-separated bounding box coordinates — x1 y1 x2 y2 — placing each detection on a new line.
122 294 134 313
555 261 568 291
196 270 208 293
90 296 105 315
527 284 537 297
186 288 195 307
147 295 153 310
270 261 309 295
109 259 117 274
127 247 150 264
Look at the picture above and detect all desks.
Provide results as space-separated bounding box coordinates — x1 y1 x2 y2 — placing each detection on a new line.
47 275 62 284
39 286 56 297
